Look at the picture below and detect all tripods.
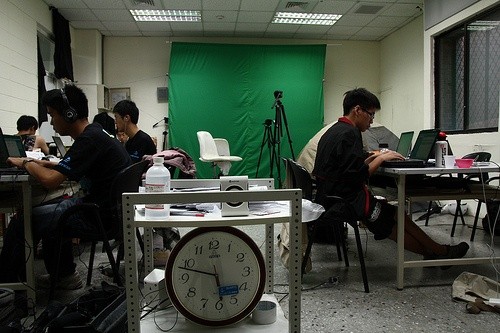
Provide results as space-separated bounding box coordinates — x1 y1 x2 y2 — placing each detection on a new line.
255 91 295 189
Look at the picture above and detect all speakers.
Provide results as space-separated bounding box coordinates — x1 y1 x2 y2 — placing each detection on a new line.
219 176 251 216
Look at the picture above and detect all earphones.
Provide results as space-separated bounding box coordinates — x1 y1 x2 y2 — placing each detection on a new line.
126 118 127 119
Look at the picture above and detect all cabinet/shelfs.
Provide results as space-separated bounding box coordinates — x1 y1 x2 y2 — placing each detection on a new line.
121 178 303 333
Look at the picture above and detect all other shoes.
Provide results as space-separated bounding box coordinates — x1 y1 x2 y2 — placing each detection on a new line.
38 268 83 291
423 242 469 269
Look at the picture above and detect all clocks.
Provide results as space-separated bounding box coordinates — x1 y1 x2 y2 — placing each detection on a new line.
164 226 267 329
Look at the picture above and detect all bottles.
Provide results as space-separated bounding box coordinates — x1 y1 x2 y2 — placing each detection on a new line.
379 143 388 153
435 132 448 168
144 157 170 219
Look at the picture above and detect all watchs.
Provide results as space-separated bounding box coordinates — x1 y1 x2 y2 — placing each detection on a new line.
22 158 34 170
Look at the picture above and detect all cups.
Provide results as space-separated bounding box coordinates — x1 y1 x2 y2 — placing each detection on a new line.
443 156 455 168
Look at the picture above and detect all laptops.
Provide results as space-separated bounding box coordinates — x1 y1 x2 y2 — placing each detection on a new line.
0 127 27 175
52 136 66 158
381 129 440 168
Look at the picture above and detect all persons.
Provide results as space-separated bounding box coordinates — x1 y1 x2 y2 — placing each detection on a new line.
0 83 158 295
12 115 50 156
297 87 470 271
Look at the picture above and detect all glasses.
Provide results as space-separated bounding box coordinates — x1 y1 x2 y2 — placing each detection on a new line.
360 107 375 118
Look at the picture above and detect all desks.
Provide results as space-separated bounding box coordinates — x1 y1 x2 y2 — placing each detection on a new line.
358 155 500 290
0 171 81 316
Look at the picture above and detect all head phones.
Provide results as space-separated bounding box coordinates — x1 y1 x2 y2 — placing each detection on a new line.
61 88 78 123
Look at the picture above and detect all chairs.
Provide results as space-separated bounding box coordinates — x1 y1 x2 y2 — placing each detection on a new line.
196 131 243 179
55 160 178 286
280 151 500 294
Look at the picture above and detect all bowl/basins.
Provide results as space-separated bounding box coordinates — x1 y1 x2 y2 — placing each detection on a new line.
455 159 474 168
